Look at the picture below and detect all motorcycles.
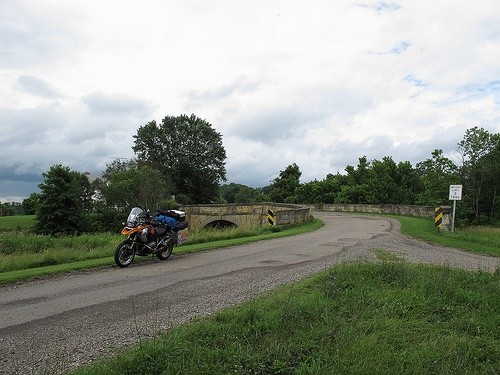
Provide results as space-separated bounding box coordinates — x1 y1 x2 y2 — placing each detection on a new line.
113 207 191 269
